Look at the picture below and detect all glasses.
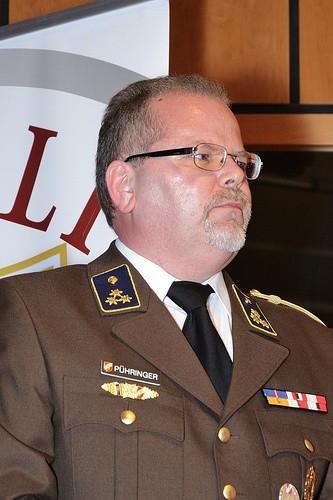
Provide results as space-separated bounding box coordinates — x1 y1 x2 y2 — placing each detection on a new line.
123 143 263 180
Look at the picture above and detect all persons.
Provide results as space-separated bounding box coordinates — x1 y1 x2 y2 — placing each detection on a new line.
0 74 333 500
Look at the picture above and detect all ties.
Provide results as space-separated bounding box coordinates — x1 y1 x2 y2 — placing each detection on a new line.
166 279 233 409
